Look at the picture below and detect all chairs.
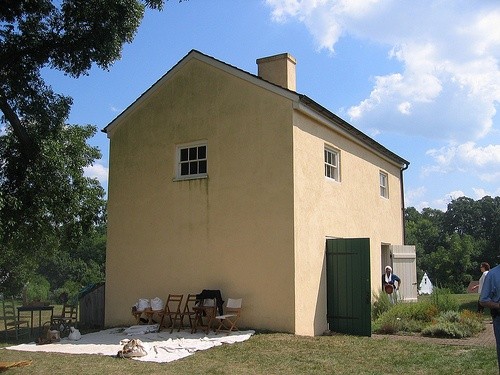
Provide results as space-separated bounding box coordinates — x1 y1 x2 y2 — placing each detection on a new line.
214 297 242 336
2 299 30 343
177 293 202 333
190 289 221 336
51 300 80 331
157 293 183 335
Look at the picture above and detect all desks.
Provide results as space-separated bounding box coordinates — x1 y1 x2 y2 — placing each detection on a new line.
139 307 161 327
16 304 55 339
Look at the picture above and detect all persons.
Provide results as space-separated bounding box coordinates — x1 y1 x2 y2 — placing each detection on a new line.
480 264 500 375
479 263 490 294
382 266 401 304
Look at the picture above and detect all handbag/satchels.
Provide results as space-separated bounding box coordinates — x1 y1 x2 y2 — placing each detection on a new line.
68 329 82 340
118 339 147 358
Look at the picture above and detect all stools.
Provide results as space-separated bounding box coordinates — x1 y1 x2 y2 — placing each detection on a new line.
49 317 72 338
